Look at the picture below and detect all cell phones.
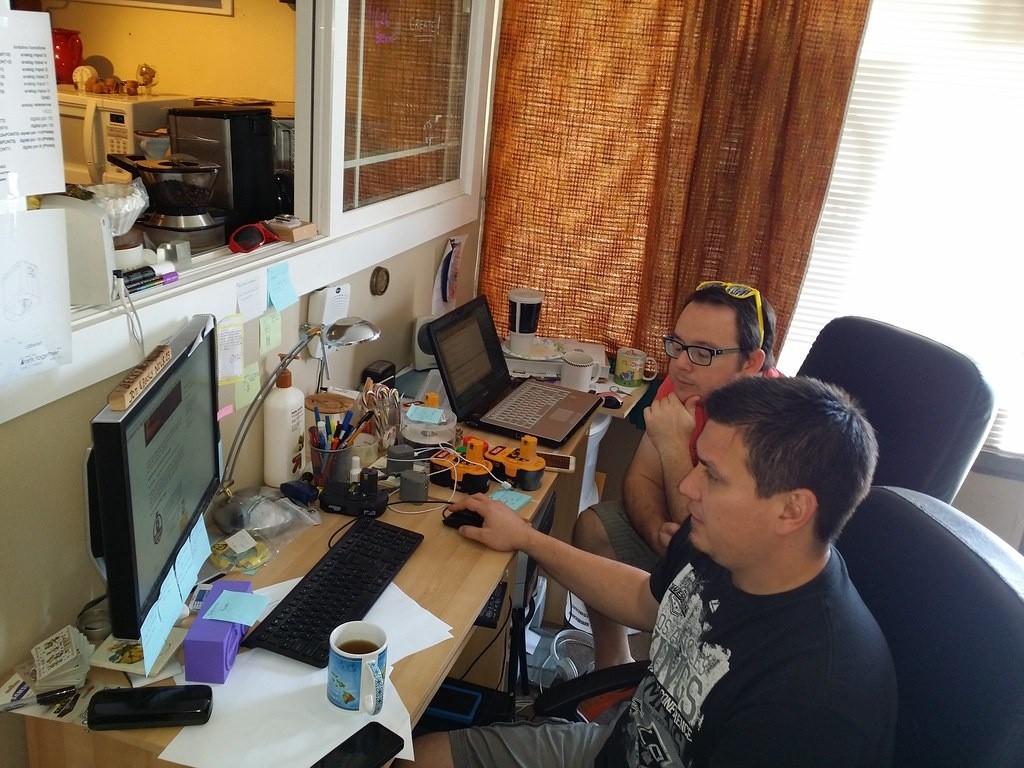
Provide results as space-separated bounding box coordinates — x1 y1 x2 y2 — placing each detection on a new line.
426 684 481 724
535 451 576 474
310 720 405 768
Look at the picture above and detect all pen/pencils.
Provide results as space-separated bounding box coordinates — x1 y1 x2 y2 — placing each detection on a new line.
309 406 373 485
0 686 76 713
514 376 560 382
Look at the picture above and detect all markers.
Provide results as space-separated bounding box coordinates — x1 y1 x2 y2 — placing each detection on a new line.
299 472 314 484
126 270 179 294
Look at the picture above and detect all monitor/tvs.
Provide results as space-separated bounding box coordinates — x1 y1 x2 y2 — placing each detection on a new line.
84 314 221 688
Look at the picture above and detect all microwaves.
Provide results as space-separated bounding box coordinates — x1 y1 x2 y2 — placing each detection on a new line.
57 83 192 184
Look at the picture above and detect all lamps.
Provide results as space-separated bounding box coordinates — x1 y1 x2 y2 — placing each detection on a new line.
212 316 380 535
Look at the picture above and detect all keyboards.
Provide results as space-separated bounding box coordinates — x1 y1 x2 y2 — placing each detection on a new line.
243 516 424 669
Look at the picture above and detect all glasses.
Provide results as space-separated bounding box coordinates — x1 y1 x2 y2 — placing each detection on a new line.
698 280 765 351
661 333 754 367
229 220 283 254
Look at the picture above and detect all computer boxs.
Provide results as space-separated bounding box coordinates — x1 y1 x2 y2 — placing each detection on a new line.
413 676 516 738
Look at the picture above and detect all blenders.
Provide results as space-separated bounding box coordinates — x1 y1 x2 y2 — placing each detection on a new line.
129 154 219 252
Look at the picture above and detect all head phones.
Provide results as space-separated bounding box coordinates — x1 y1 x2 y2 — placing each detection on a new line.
550 629 596 680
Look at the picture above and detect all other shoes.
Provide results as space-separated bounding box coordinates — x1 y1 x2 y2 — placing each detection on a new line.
576 684 640 722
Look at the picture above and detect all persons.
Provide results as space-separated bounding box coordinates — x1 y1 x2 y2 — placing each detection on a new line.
390 376 897 768
577 280 777 724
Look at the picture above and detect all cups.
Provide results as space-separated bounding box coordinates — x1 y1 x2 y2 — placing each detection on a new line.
309 441 353 493
507 288 543 356
560 351 601 393
614 348 658 387
326 620 388 716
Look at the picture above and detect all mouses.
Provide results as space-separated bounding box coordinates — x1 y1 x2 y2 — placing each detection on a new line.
443 509 484 530
596 391 624 409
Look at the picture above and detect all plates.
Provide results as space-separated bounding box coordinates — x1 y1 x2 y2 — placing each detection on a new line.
502 336 568 360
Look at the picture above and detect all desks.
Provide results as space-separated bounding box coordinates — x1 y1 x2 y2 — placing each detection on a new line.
22 351 653 768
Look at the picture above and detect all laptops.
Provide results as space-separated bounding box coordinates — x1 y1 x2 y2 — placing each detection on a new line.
426 293 605 446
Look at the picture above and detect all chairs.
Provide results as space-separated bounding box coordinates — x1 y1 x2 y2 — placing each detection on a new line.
793 316 995 507
536 485 1024 768
